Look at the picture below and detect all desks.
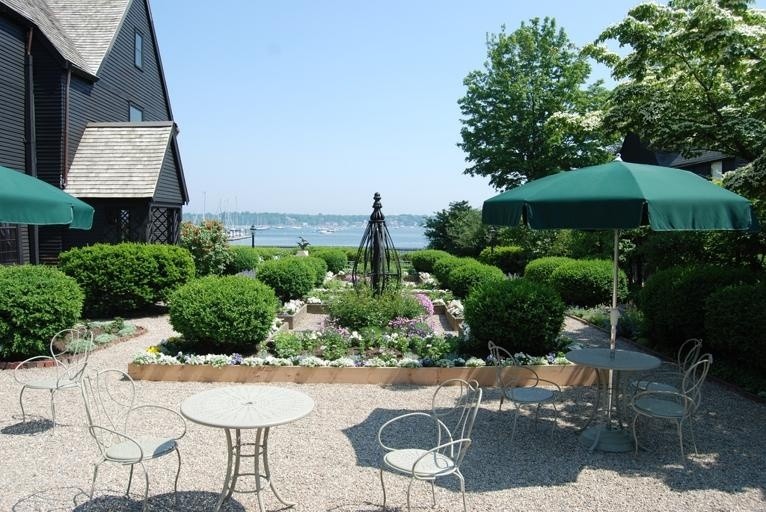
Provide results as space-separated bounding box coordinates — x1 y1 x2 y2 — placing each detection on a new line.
564 348 662 453
180 384 313 512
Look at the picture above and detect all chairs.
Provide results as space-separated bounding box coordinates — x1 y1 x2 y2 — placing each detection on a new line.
378 341 562 512
13 328 187 512
628 337 716 470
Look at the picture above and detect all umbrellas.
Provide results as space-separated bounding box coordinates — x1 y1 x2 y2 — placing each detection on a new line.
480 153 752 427
0 163 96 230
